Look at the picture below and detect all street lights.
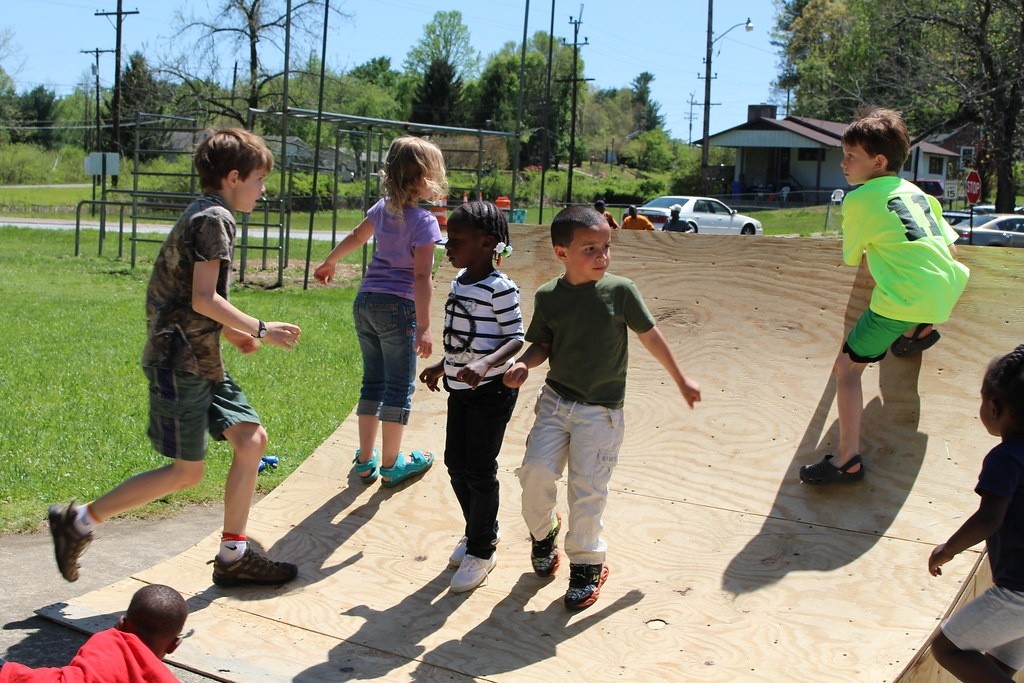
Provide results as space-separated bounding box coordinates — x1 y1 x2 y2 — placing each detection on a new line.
699 16 754 194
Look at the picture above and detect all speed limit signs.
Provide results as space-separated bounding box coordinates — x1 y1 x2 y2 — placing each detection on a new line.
944 181 959 200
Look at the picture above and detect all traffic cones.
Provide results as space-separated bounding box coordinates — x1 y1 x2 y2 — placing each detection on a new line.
461 193 468 204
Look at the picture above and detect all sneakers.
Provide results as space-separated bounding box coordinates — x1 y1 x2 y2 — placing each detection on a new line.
450 549 498 592
206 547 298 586
563 561 609 610
49 498 93 583
529 512 563 579
448 527 500 566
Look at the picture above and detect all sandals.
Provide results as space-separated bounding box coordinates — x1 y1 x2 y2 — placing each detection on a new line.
890 322 940 357
379 450 433 488
352 448 379 484
800 454 864 483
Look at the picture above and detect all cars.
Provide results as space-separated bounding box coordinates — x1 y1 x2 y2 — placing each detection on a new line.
950 213 1024 248
622 194 763 236
960 205 1024 214
942 210 977 226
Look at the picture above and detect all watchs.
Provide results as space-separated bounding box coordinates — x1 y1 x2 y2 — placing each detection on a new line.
252 320 267 339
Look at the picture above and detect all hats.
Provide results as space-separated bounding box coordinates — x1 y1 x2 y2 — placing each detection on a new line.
592 200 609 206
670 204 681 211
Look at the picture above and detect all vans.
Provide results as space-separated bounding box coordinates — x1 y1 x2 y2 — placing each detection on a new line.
904 179 946 197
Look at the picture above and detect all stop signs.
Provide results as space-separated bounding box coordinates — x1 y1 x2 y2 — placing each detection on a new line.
964 170 981 205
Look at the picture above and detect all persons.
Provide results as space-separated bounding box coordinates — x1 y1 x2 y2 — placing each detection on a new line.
662 204 695 233
313 138 447 487
49 127 302 588
928 344 1024 683
503 203 701 608
621 205 655 230
0 584 187 683
595 199 620 229
418 201 524 593
798 109 969 487
